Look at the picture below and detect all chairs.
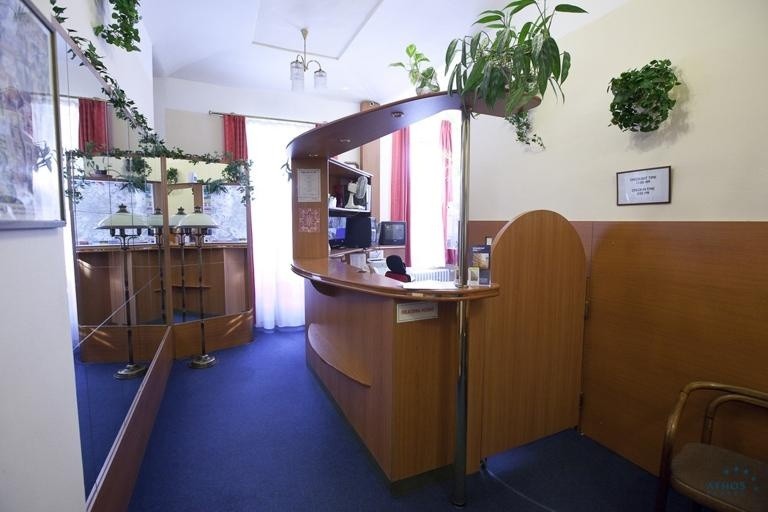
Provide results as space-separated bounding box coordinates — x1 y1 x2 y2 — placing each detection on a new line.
654 371 768 511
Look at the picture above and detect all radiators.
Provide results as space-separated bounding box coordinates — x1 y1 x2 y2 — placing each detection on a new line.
400 265 452 283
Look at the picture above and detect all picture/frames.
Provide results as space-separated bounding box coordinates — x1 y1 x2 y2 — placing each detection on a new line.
614 164 671 207
0 0 68 233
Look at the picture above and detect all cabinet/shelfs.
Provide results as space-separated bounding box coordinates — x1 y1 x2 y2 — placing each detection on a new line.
283 155 376 260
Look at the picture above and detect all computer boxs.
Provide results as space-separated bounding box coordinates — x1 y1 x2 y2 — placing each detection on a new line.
345 217 376 248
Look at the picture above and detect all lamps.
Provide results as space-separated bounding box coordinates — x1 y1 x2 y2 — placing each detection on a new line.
287 28 327 94
92 202 152 381
166 205 189 322
146 207 169 327
175 204 224 369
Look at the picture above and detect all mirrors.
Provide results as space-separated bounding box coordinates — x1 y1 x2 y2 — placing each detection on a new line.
52 25 168 505
167 156 254 327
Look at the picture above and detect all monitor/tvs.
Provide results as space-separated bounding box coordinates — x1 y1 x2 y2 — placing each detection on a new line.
380 221 405 245
328 215 348 248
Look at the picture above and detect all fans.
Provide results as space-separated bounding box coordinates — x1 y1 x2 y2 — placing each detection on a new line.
340 174 369 211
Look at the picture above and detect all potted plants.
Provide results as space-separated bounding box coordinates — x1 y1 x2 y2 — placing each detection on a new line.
391 43 441 97
440 1 592 155
599 51 684 135
